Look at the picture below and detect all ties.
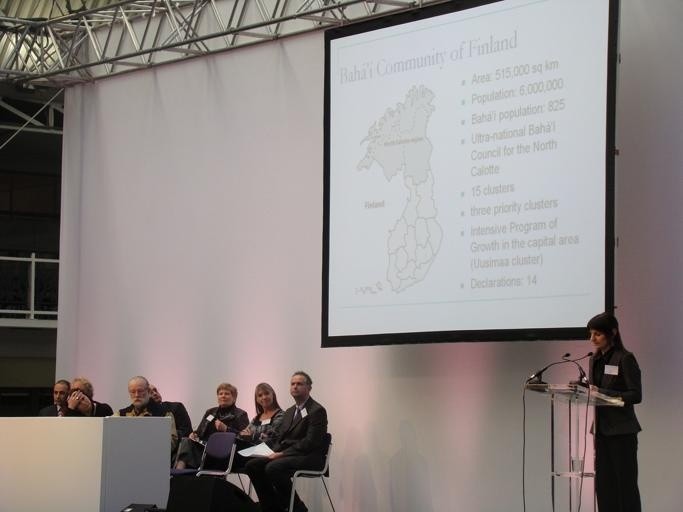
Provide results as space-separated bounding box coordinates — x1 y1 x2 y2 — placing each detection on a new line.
292 407 299 420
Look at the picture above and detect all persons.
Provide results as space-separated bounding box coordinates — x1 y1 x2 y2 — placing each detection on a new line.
586 311 642 512
39 375 327 512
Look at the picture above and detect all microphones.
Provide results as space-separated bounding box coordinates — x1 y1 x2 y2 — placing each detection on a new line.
562 353 588 389
529 352 593 384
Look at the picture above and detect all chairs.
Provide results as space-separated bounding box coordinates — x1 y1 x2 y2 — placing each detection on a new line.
284 433 339 512
171 430 236 482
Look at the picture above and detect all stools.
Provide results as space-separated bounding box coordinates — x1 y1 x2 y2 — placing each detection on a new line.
243 471 263 505
